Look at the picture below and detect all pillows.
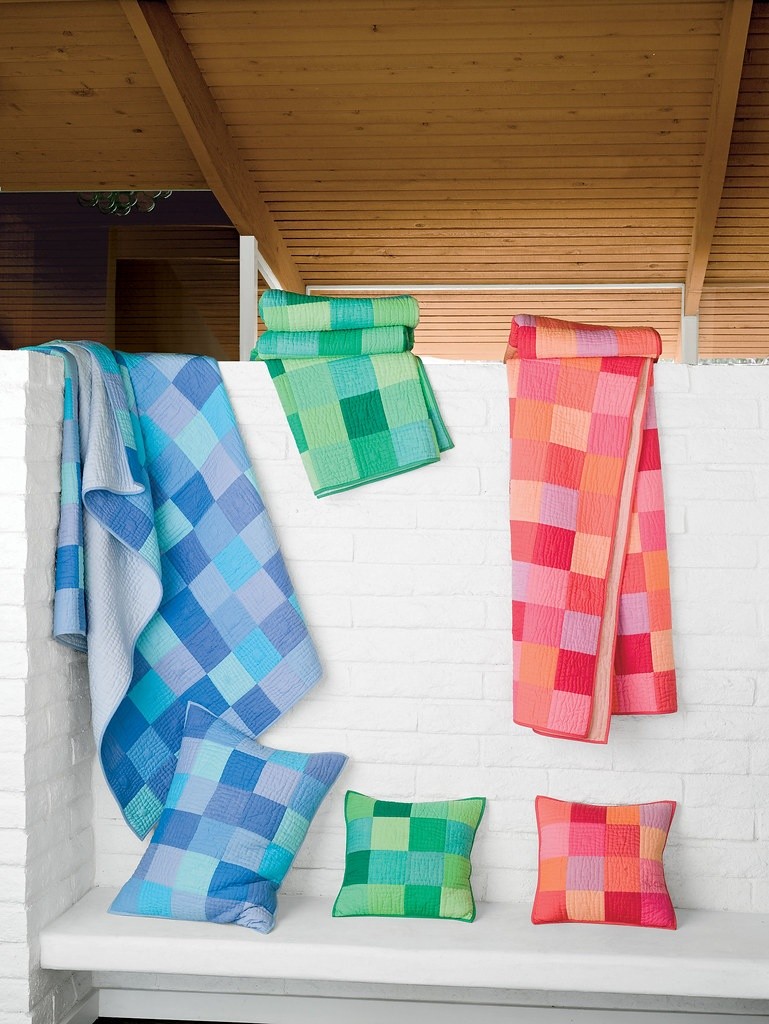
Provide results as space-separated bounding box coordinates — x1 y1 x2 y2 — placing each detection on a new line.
332 791 486 922
103 700 348 934
531 795 679 929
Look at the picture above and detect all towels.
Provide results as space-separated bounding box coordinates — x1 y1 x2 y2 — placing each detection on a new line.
249 291 458 498
502 313 679 745
22 338 323 843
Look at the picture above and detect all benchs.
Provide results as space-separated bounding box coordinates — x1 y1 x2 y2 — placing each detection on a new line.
39 885 769 1016
